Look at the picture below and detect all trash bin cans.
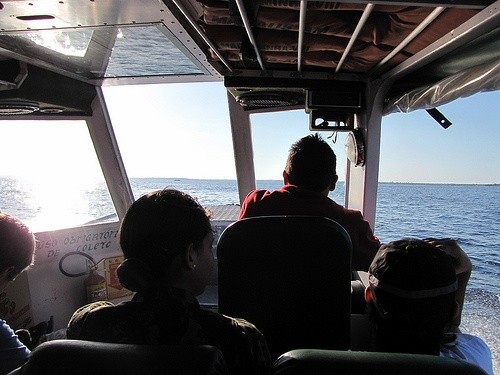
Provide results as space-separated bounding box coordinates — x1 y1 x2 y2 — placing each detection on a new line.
59 252 108 303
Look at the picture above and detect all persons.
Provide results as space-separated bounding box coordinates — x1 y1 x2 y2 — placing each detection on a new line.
356 237 494 375
0 216 41 375
65 189 274 374
241 133 381 315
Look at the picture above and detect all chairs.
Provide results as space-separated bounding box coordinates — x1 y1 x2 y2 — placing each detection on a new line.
216 215 353 353
19 339 226 375
